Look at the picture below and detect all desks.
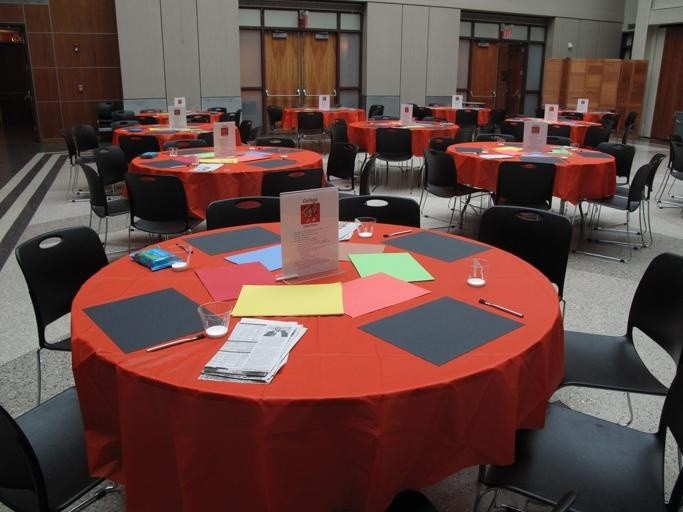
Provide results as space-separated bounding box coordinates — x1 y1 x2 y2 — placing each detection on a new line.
70 220 564 510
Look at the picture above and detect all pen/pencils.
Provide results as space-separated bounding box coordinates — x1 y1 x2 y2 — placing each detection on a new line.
479 298 524 318
176 243 193 254
146 333 207 352
384 230 412 237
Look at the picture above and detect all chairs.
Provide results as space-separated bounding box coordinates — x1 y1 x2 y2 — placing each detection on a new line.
655 111 683 209
262 107 637 193
475 351 682 512
477 207 573 321
558 252 682 426
63 104 253 201
1 384 121 512
15 225 108 405
83 139 381 254
419 143 665 263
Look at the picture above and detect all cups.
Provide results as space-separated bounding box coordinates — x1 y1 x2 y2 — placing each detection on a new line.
368 118 375 123
247 140 257 150
191 157 200 166
497 138 505 145
440 121 447 127
279 147 291 157
481 146 488 153
570 143 578 150
169 147 178 156
